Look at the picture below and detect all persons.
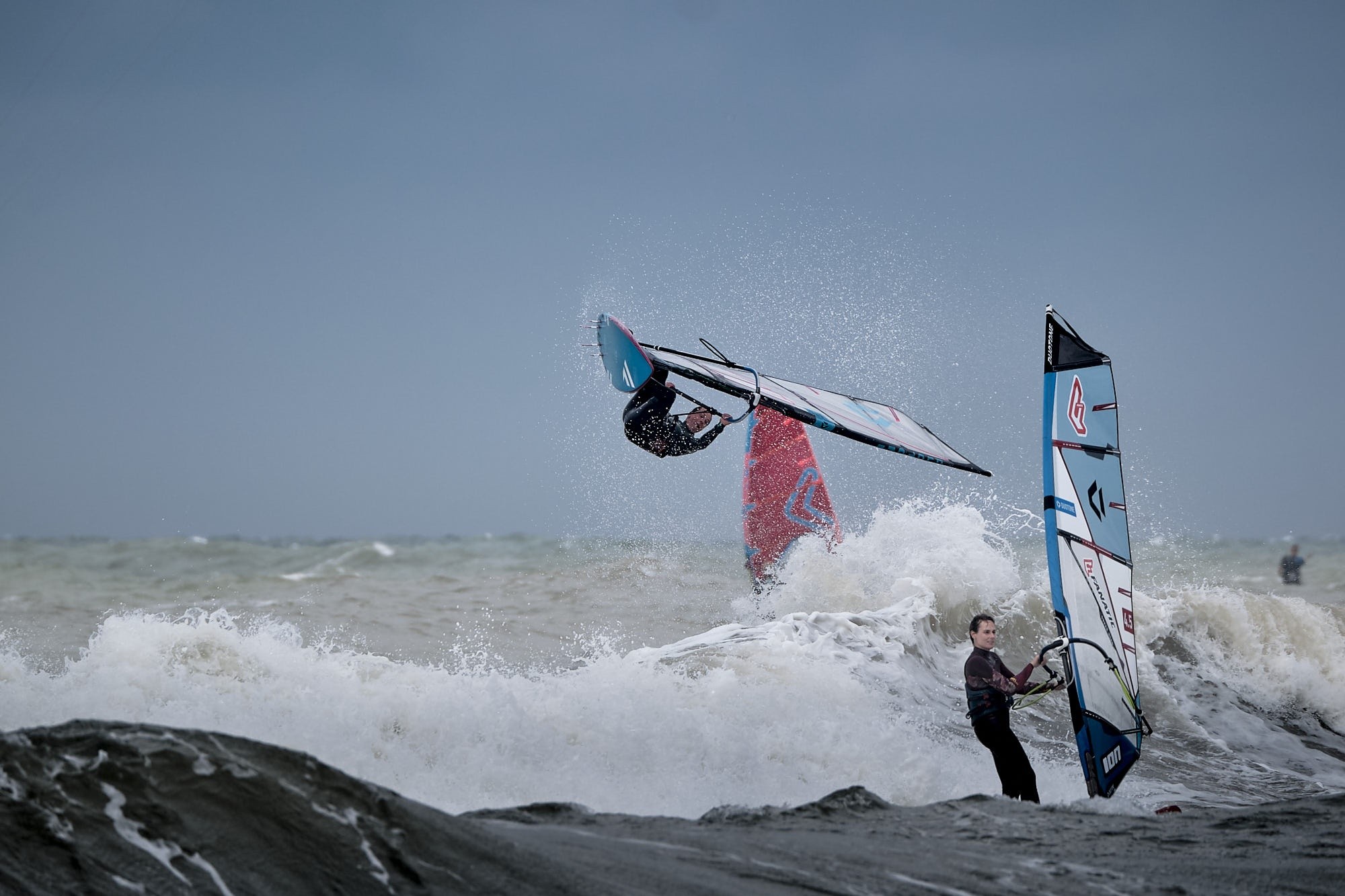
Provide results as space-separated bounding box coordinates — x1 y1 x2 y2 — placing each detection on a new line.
1278 543 1305 586
623 366 733 458
964 613 1054 804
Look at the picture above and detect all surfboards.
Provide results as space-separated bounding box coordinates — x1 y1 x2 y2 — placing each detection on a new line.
578 313 655 394
